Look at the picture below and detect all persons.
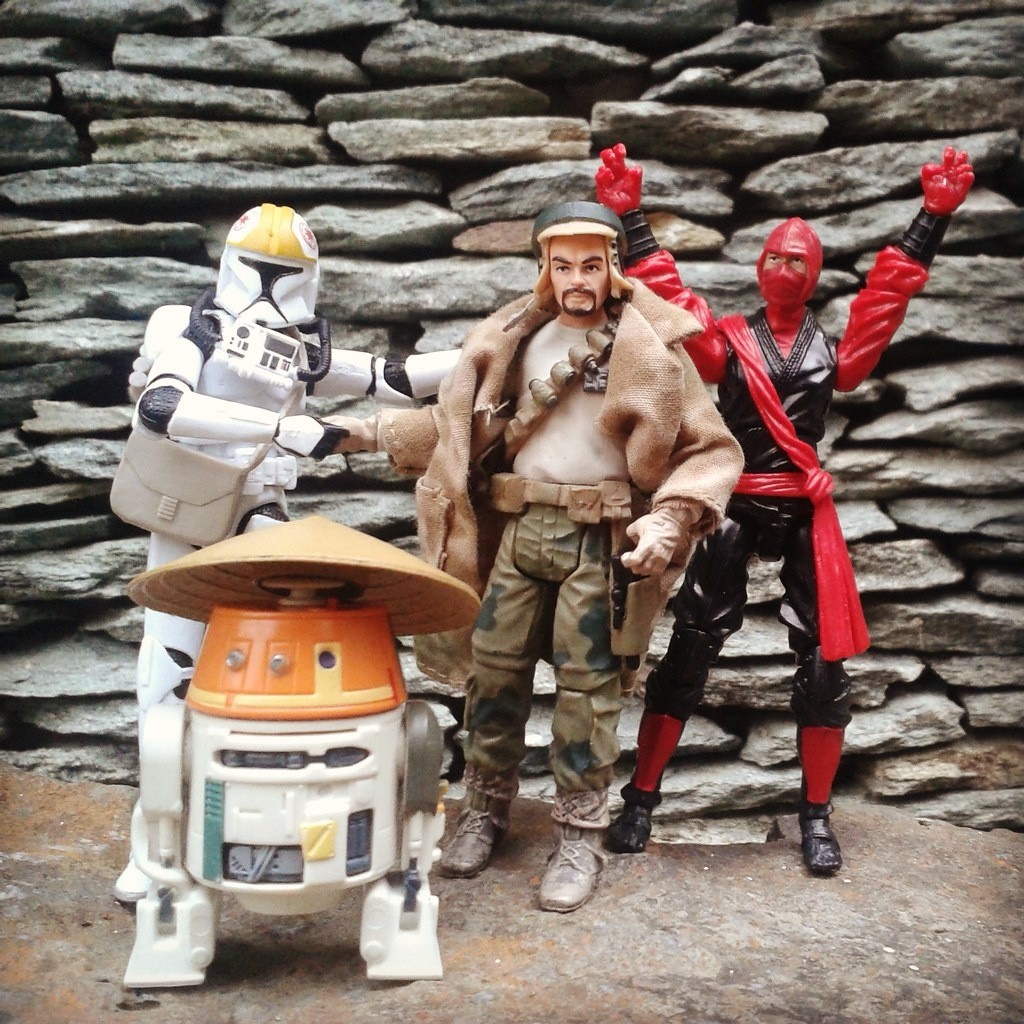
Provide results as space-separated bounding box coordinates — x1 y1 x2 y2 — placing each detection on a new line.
313 200 747 909
117 203 460 901
591 145 974 880
